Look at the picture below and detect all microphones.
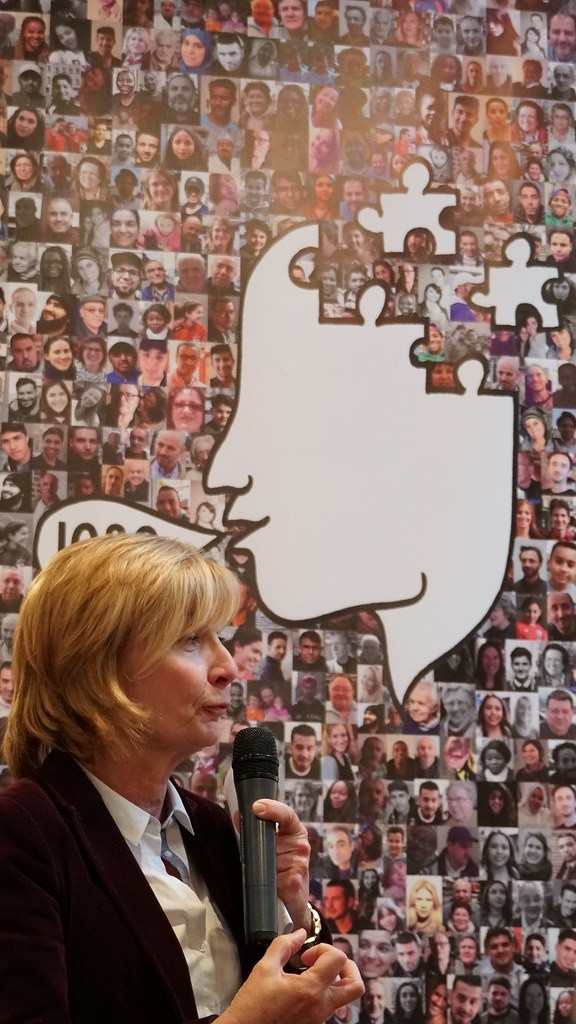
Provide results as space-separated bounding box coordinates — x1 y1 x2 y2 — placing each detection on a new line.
231 727 279 950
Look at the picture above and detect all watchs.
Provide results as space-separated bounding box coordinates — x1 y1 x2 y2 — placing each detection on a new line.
302 902 321 943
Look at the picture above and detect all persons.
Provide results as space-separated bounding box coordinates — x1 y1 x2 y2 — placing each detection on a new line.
9 286 37 334
209 344 235 388
123 460 148 503
151 430 186 480
138 340 168 388
69 426 101 470
240 220 270 258
75 295 105 337
0 534 366 1024
209 298 237 344
174 255 208 299
260 632 289 689
207 218 237 255
75 338 108 383
30 428 67 471
0 0 576 1024
108 302 138 337
167 343 204 386
143 303 169 339
109 251 140 301
